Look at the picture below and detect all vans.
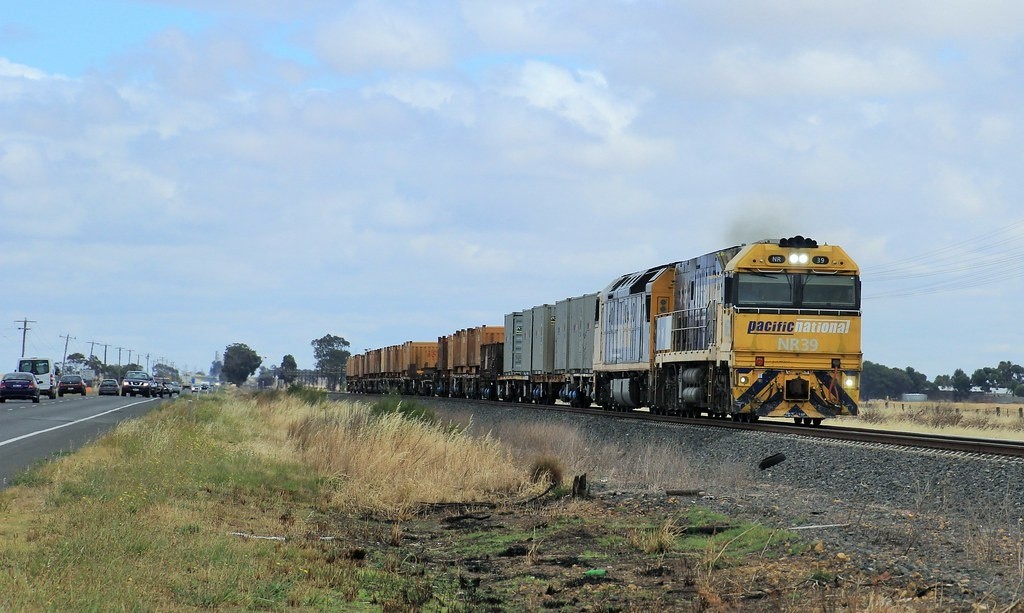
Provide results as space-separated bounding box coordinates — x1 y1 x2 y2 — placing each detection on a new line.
16 355 57 399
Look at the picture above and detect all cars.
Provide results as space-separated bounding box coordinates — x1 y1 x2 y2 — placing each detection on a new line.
120 370 208 397
0 371 40 404
57 374 86 398
98 377 120 395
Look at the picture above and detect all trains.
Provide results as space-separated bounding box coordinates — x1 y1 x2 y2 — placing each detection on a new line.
341 236 864 431
